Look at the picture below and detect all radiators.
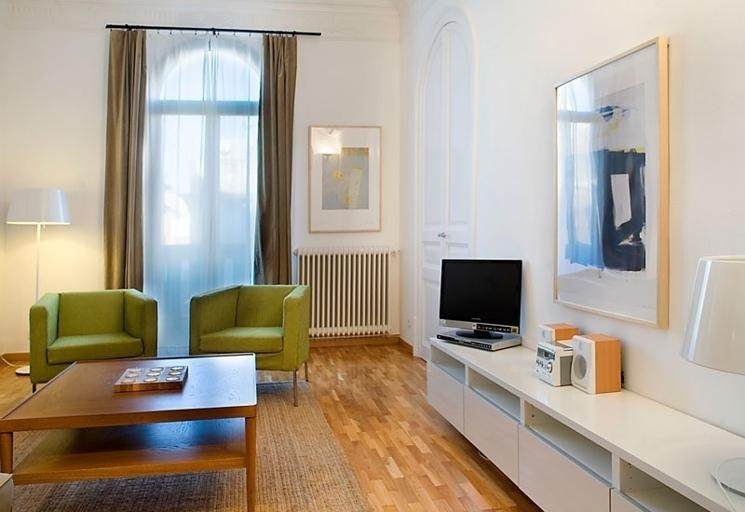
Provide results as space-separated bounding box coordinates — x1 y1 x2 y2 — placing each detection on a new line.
293 243 403 339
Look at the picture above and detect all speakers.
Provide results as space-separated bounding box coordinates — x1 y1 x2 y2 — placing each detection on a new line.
539 322 580 342
570 333 622 395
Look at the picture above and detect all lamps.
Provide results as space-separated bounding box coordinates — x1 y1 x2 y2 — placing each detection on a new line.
679 257 744 495
4 185 72 377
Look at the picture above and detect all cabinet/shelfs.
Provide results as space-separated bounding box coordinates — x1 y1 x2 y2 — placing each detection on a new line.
425 331 745 512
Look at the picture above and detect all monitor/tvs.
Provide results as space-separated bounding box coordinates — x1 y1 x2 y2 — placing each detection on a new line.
439 258 523 339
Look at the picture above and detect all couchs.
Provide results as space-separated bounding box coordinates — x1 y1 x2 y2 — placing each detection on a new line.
185 282 313 408
28 286 159 395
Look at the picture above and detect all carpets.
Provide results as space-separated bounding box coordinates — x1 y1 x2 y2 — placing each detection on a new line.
0 377 369 512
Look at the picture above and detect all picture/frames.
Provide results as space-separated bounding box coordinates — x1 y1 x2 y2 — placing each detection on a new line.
550 36 670 330
308 121 383 234
